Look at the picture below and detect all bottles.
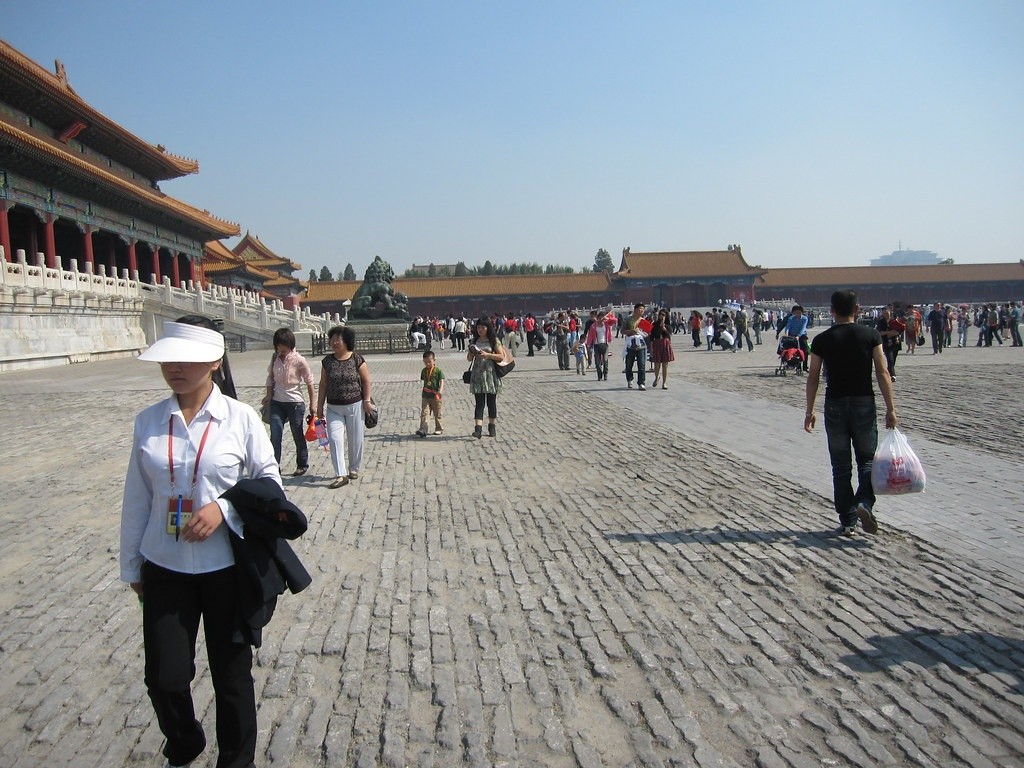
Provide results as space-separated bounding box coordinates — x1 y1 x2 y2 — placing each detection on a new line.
314 420 330 446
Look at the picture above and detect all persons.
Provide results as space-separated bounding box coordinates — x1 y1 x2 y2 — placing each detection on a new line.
550 314 570 371
415 351 445 437
872 301 1023 382
573 333 588 375
119 315 284 768
803 286 898 536
259 327 315 476
620 303 649 390
687 298 878 372
650 309 675 390
587 311 618 381
407 308 687 369
467 316 505 439
316 326 374 489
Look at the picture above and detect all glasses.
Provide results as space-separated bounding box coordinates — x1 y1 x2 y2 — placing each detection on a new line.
658 312 665 317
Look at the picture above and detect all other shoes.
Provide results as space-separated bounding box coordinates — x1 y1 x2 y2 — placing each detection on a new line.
416 429 443 438
526 349 668 390
329 469 358 488
857 504 878 535
841 524 856 534
933 341 1023 356
293 467 306 476
891 376 895 381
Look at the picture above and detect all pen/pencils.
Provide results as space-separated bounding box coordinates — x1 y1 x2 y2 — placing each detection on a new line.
176 494 182 542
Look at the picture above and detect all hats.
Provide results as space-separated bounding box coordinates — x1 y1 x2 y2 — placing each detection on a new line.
136 322 225 362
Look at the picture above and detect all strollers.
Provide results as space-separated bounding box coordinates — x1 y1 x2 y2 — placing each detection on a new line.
775 336 804 377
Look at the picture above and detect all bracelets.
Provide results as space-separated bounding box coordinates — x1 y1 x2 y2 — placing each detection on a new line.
488 353 492 358
363 400 370 402
806 411 815 415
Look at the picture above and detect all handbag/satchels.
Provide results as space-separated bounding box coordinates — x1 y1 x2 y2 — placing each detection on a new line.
364 398 379 428
492 337 516 378
916 332 925 346
958 326 963 334
543 322 553 333
261 398 289 425
463 370 472 384
597 343 608 353
505 325 513 333
871 427 925 494
305 414 319 441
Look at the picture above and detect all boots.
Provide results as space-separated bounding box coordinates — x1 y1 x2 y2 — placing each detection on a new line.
488 423 496 436
473 425 482 439
